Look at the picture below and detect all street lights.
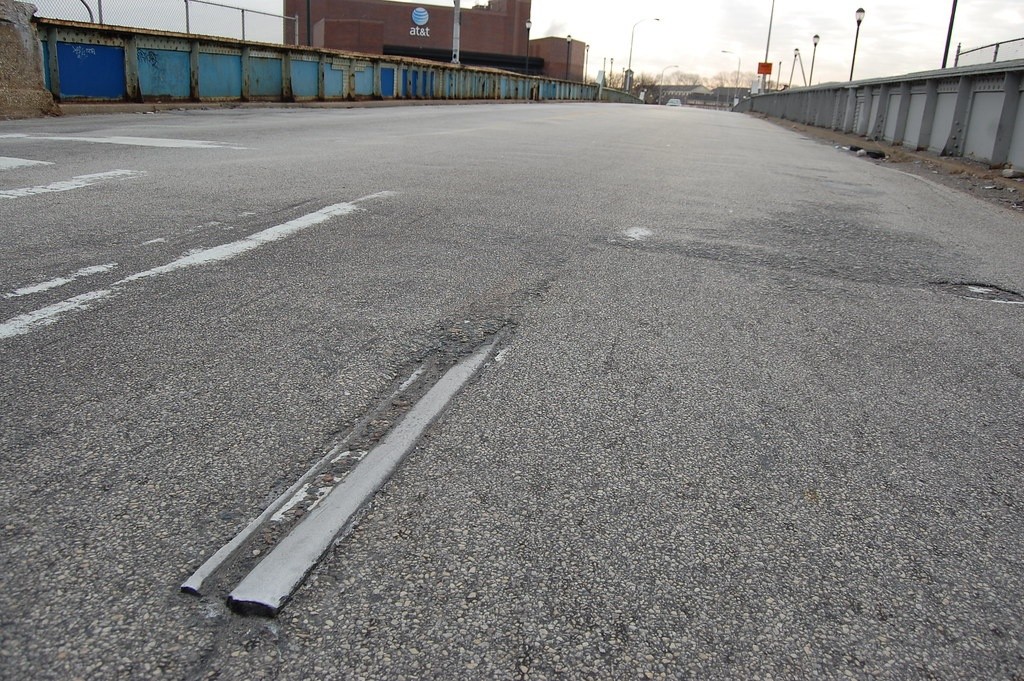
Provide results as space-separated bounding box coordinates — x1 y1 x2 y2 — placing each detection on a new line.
609 57 615 89
566 35 572 80
603 57 607 87
525 19 532 75
659 65 680 105
721 50 742 98
627 18 660 94
585 44 589 84
808 33 820 87
787 48 799 87
849 6 866 81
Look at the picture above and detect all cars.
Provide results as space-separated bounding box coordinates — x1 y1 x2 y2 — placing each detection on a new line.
666 99 681 106
